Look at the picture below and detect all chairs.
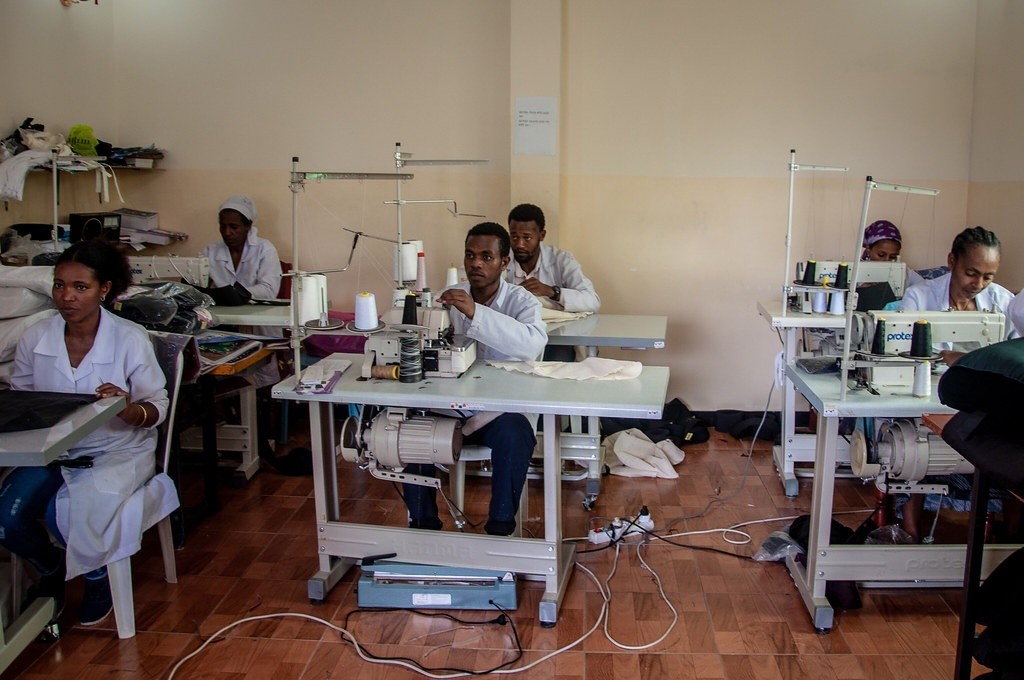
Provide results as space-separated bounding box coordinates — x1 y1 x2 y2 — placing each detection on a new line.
12 328 184 639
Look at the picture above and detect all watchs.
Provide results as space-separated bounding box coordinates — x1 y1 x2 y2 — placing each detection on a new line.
549 285 560 301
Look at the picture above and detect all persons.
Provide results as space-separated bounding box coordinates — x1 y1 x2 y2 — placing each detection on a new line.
501 203 603 472
401 224 538 537
0 238 170 626
890 225 1018 545
180 194 282 466
860 220 924 302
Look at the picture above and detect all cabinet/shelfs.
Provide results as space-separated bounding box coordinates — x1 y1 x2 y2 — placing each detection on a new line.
25 149 166 246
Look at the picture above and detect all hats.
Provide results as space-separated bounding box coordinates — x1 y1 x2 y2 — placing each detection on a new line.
219 194 256 222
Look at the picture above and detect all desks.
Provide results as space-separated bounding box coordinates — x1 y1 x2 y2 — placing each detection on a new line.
922 412 1024 680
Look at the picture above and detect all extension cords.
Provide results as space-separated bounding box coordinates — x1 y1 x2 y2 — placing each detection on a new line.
589 517 655 544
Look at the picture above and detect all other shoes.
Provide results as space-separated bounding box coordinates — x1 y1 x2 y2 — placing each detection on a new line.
80 579 113 626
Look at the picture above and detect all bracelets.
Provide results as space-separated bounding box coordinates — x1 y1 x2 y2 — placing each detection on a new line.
129 404 147 429
